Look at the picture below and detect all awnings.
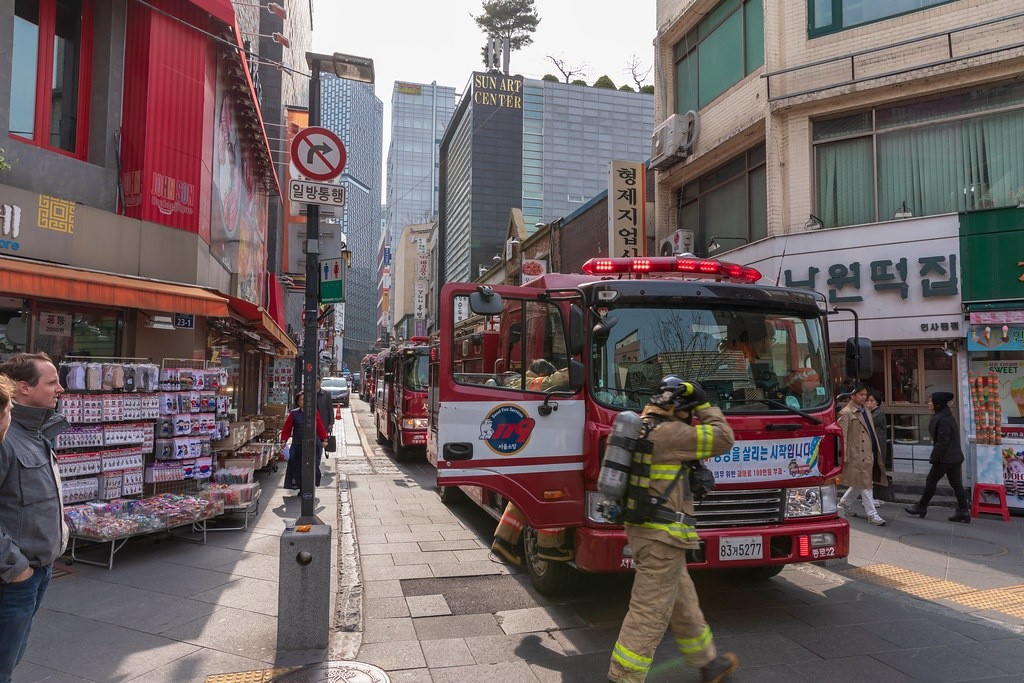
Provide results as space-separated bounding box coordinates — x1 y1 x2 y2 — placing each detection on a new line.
0 255 229 317
213 291 298 354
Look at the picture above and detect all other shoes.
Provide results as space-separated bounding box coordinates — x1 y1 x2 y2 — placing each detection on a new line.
537 546 575 562
491 537 521 567
702 651 740 683
298 491 302 496
862 499 885 508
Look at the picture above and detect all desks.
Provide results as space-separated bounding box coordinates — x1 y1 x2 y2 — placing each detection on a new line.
69 511 225 570
192 490 263 532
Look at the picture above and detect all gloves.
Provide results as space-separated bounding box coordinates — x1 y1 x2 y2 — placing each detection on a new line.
660 381 705 412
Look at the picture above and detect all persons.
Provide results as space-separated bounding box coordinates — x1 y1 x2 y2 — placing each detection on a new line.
0 373 17 443
490 359 576 566
718 317 777 399
606 372 739 683
836 378 886 526
904 392 971 523
0 352 70 683
280 375 334 496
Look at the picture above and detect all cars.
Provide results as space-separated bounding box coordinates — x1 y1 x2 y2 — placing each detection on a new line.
319 377 351 407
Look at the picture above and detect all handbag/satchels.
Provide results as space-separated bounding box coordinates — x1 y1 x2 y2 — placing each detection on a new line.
689 465 715 497
324 430 336 459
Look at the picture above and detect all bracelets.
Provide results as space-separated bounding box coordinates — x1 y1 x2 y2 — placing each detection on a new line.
281 442 285 443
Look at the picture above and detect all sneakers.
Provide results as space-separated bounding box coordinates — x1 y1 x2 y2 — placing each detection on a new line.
836 502 857 517
868 515 886 526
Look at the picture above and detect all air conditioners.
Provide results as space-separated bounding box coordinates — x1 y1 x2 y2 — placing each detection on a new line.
660 229 696 258
649 113 691 170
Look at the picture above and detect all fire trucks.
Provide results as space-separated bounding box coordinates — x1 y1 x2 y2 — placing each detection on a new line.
425 253 878 596
359 353 378 401
372 334 431 460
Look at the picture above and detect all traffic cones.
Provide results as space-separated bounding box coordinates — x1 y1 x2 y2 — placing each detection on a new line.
334 403 343 419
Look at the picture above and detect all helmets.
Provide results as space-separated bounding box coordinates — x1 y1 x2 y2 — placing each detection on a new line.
655 374 688 395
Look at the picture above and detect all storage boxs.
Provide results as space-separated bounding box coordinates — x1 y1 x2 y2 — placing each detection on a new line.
213 402 290 470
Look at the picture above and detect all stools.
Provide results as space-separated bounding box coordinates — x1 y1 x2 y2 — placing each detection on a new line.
972 482 1012 522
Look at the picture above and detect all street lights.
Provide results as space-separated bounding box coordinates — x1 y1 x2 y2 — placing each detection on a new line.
296 48 374 527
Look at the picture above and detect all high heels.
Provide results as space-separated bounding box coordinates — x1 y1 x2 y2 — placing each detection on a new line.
904 500 927 518
948 508 971 523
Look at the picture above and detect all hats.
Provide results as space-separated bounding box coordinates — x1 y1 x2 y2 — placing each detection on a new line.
932 392 954 411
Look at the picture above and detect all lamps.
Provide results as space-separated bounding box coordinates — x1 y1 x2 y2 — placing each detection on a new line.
247 60 292 75
232 2 287 20
241 31 288 49
805 214 824 230
894 201 913 219
707 237 748 253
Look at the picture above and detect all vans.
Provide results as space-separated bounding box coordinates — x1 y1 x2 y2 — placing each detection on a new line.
352 373 360 392
